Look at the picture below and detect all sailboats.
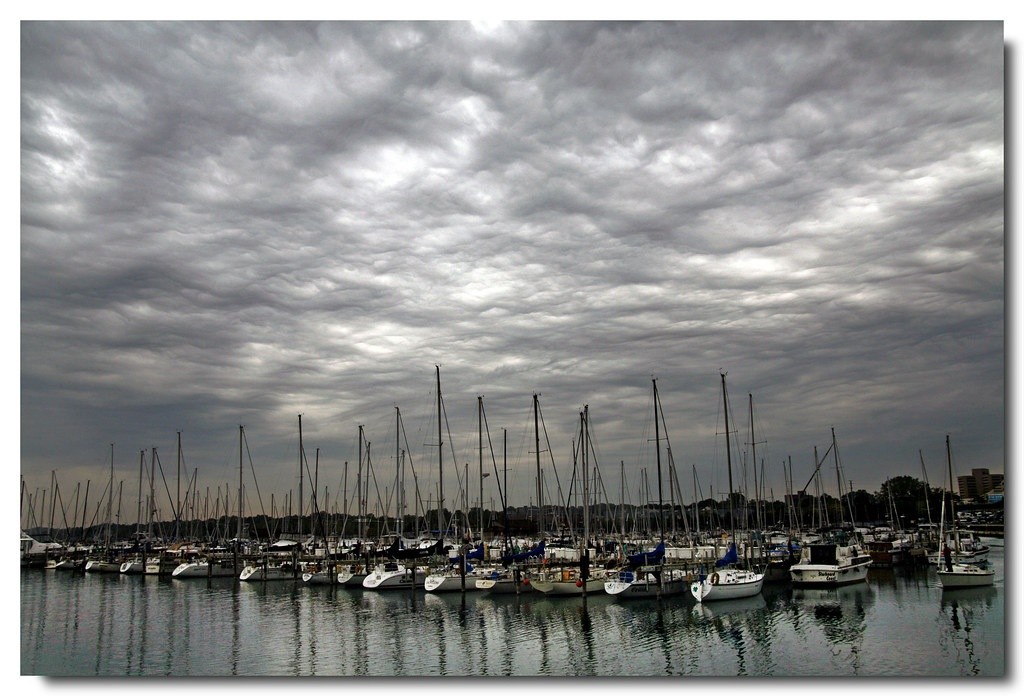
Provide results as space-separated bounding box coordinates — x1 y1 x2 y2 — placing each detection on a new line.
688 368 772 598
935 434 996 587
21 361 943 603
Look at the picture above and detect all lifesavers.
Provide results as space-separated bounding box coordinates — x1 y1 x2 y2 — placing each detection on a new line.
710 573 719 586
356 565 362 574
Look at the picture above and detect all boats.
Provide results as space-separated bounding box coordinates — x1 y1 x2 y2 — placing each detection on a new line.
927 528 992 565
